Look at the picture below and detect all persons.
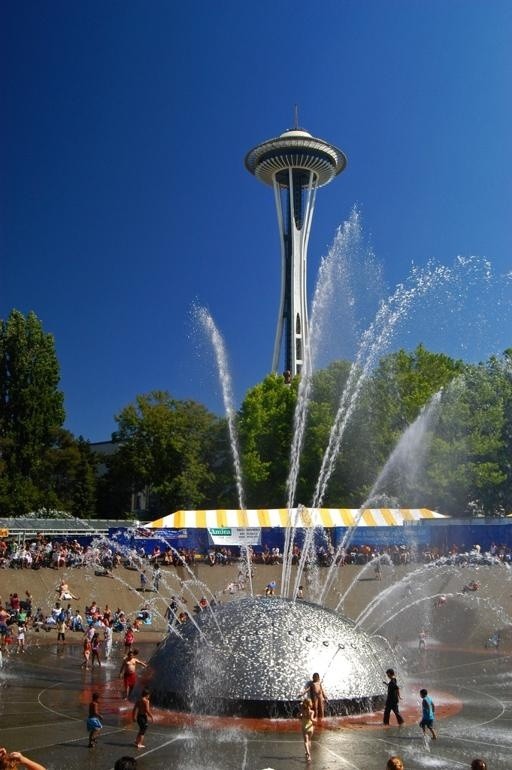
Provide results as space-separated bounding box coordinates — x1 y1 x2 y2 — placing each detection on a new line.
418 689 438 740
131 687 155 750
470 758 487 770
0 746 48 770
85 692 105 748
298 672 328 721
114 755 137 770
385 755 404 770
2 528 512 671
381 669 405 727
118 650 149 701
295 697 318 761
